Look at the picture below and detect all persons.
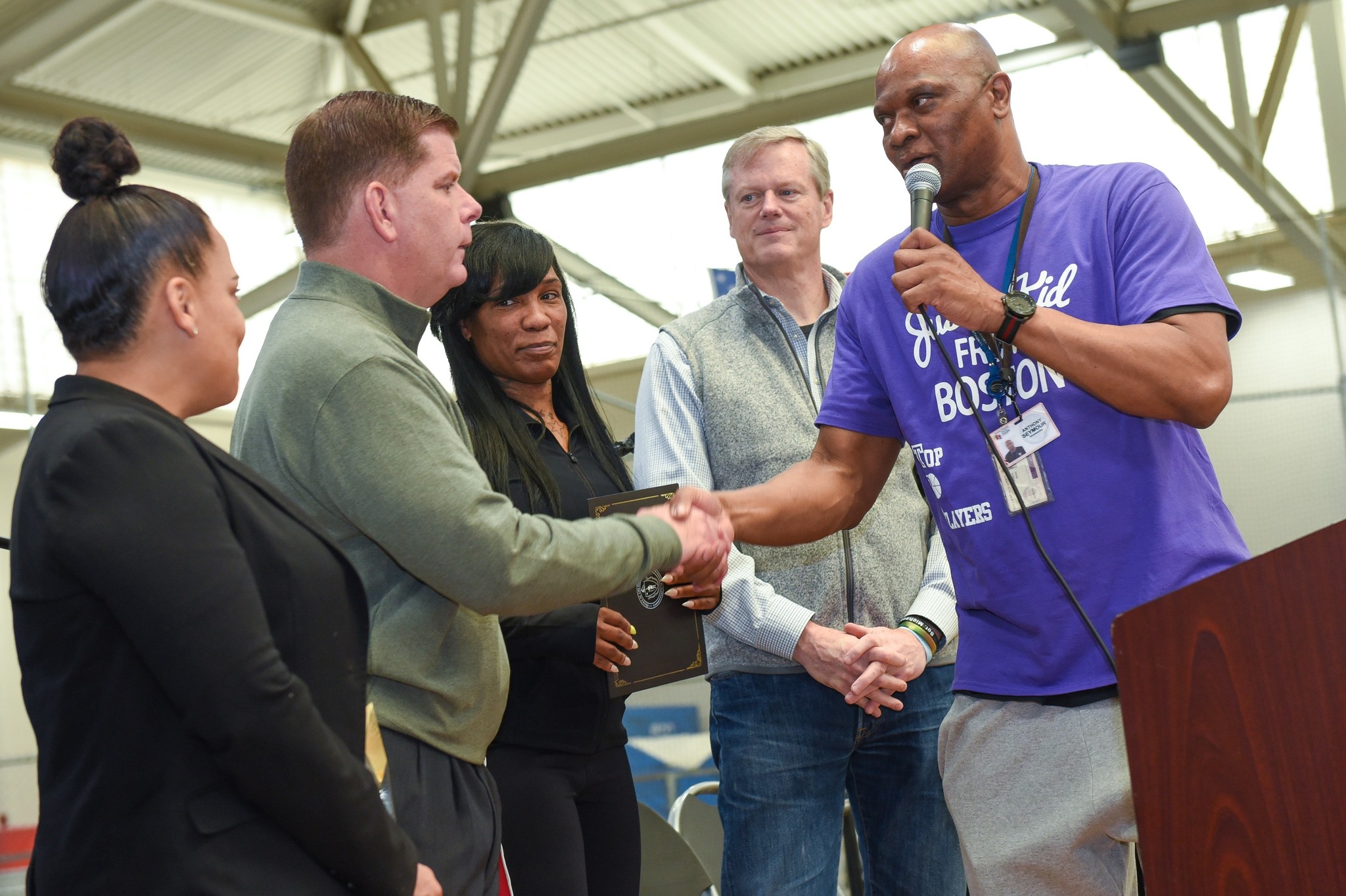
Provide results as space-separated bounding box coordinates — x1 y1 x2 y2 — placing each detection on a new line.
634 125 966 896
669 23 1254 896
229 91 721 896
9 117 442 896
430 218 723 896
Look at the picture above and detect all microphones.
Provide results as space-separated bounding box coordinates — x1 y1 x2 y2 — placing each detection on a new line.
904 163 942 233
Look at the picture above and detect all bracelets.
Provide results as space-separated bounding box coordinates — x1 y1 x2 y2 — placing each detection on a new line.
898 617 939 664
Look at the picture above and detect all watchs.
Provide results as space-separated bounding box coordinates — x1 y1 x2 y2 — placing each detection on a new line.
993 290 1037 348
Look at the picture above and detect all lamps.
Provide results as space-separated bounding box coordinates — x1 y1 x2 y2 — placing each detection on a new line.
1119 33 1164 71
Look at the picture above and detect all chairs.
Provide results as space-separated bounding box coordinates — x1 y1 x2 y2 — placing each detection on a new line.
639 779 725 896
